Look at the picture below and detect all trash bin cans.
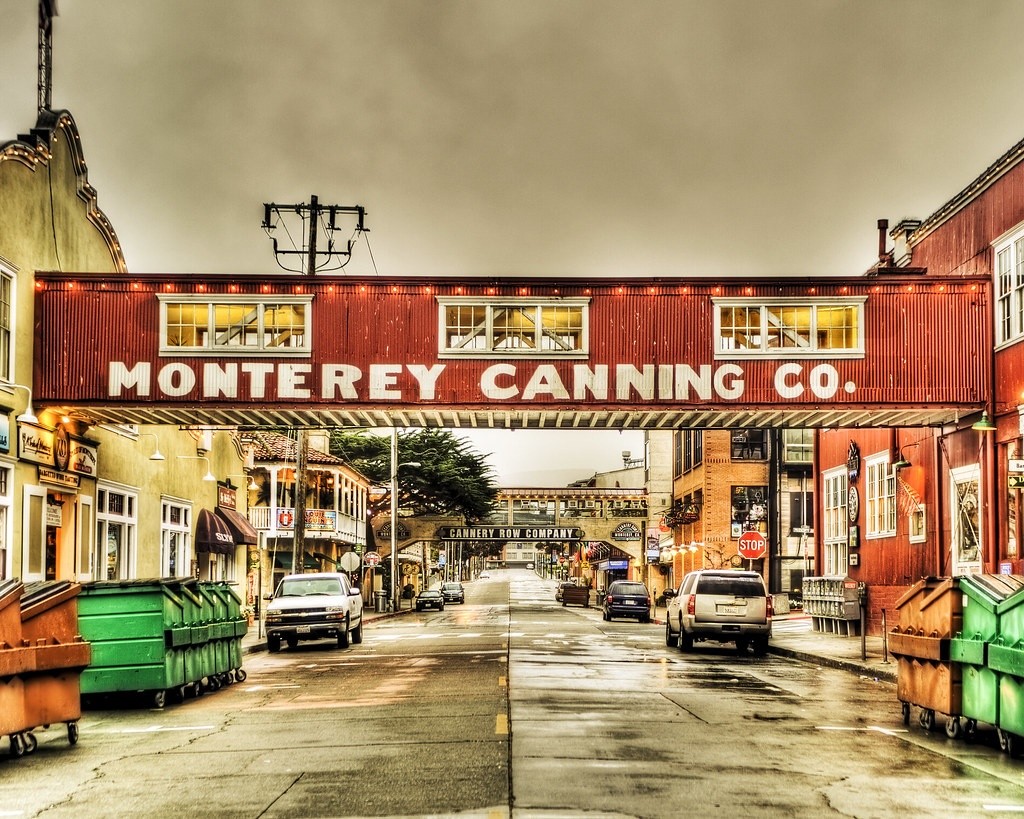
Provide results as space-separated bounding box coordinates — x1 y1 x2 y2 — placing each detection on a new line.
0 577 91 756
454 572 458 581
556 570 560 579
888 573 963 738
78 575 248 710
950 574 1024 756
373 590 387 612
596 590 606 606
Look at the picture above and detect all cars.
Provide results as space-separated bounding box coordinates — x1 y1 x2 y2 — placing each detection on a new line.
601 579 651 623
415 590 444 611
526 564 534 570
262 573 364 652
555 582 576 601
480 571 489 578
439 582 465 604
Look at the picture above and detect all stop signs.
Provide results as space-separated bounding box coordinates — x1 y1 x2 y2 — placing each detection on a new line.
738 531 766 560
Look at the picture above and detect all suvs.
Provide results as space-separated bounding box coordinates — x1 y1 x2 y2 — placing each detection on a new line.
663 569 773 653
